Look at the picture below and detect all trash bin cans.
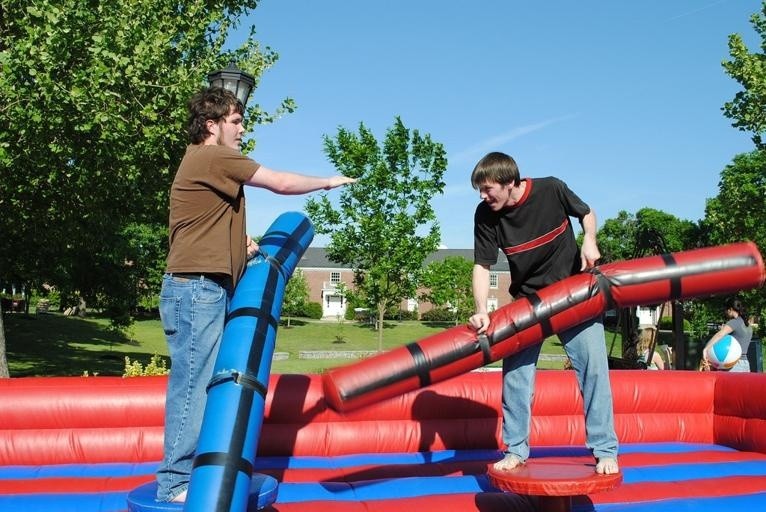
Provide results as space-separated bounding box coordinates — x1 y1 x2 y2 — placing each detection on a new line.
12 299 25 312
746 339 766 372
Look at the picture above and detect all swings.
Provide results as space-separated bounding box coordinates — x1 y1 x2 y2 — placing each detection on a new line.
605 304 667 369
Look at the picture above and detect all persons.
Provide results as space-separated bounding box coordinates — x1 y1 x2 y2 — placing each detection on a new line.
701 296 753 372
154 86 357 505
624 325 672 370
468 153 621 475
